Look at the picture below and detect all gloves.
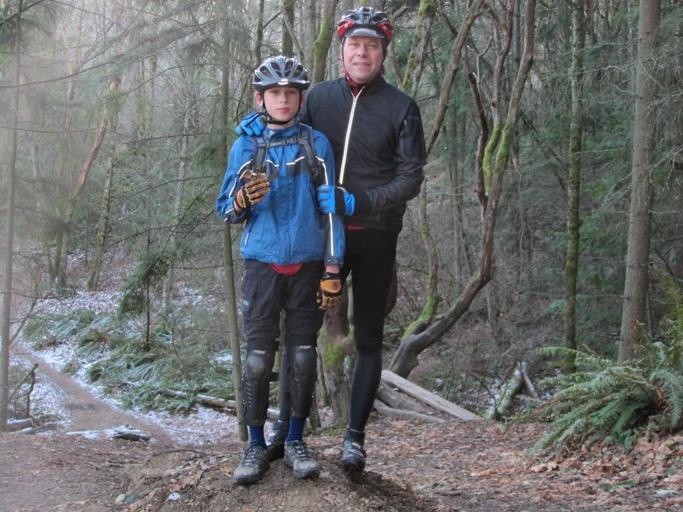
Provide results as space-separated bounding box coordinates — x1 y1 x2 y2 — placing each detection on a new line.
312 184 355 218
233 111 265 138
315 271 342 312
232 175 269 218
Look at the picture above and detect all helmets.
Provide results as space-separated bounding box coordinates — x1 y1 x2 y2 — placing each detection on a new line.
250 54 310 93
336 6 392 47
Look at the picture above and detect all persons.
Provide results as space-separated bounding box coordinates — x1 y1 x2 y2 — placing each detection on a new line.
267 8 426 480
216 55 345 484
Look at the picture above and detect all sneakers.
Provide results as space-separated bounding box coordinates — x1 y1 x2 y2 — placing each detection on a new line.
337 427 367 472
231 439 270 485
243 418 290 463
281 439 321 479
